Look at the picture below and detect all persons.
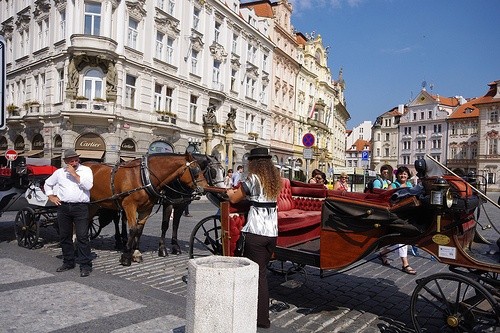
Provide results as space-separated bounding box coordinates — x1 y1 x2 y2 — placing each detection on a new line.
215 166 247 217
309 168 350 191
228 147 283 328
43 148 94 277
364 164 421 274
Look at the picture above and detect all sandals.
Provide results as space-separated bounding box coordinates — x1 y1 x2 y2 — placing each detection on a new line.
377 253 390 265
402 265 417 274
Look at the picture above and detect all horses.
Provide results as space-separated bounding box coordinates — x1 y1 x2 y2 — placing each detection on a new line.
103 149 231 257
80 151 209 268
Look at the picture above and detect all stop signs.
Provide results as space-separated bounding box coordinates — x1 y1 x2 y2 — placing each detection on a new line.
5 150 18 161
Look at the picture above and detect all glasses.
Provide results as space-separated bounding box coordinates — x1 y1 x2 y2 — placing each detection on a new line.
316 177 323 180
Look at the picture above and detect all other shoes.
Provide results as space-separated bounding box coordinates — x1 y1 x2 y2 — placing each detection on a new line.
184 213 193 217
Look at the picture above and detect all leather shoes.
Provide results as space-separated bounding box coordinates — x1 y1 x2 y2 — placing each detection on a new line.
56 266 69 271
81 268 90 276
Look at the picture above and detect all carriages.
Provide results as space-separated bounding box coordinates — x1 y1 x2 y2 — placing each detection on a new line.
0 150 227 267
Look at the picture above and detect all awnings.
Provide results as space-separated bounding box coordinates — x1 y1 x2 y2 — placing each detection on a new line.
0 150 24 156
120 157 135 163
19 150 45 158
75 150 106 161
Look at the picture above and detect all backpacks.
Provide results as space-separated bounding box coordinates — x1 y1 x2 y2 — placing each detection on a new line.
364 177 384 192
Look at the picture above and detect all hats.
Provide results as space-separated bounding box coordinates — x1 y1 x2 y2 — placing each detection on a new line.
247 147 272 160
61 148 81 159
340 171 348 177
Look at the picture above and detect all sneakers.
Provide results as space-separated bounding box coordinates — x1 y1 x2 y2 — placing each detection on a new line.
431 255 434 261
410 245 417 256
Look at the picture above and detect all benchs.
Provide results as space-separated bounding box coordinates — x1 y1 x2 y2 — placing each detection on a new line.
232 178 323 250
422 160 488 250
